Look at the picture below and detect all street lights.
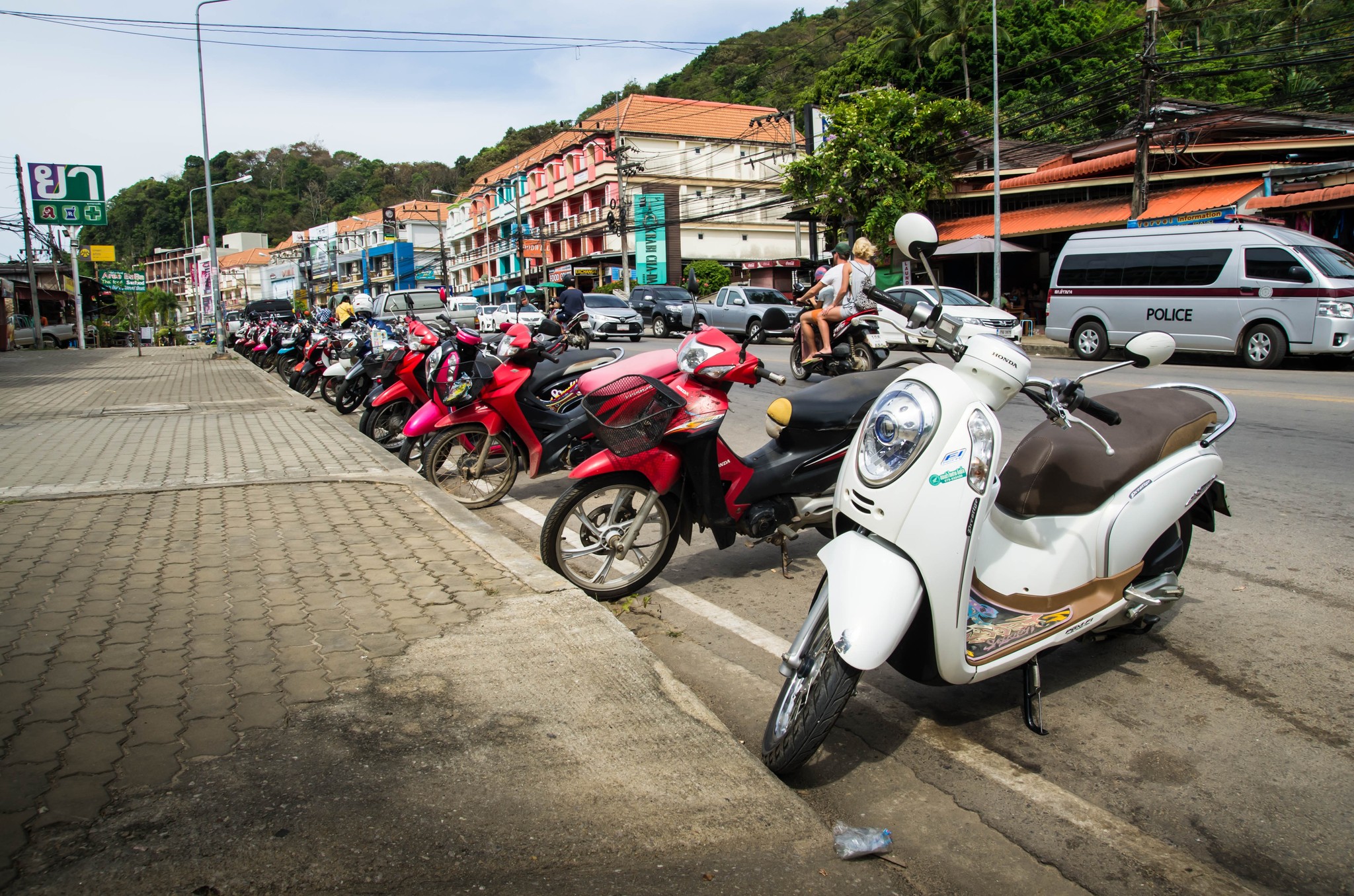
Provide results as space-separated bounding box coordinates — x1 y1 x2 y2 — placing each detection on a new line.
188 175 254 341
351 216 400 290
259 252 313 311
432 189 493 305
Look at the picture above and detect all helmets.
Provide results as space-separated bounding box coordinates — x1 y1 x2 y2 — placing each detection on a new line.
562 274 575 286
304 311 310 317
353 293 374 314
813 265 832 282
248 311 259 321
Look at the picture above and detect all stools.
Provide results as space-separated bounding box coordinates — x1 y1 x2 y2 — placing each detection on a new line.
1020 307 1043 337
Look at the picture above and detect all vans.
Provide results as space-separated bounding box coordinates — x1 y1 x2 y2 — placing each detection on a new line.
1044 212 1354 368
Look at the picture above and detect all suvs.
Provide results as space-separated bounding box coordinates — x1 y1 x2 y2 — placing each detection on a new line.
241 298 296 325
184 333 199 345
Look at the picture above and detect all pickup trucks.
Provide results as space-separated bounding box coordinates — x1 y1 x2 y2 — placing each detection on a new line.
680 284 806 344
623 285 691 338
373 289 479 332
6 312 78 349
223 310 245 348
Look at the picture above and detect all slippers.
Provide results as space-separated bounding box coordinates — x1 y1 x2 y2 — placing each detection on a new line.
802 358 819 366
812 351 832 358
560 336 569 343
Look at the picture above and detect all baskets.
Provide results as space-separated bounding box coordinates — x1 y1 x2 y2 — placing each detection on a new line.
332 338 365 359
276 332 302 349
581 374 687 458
361 349 405 379
430 361 494 407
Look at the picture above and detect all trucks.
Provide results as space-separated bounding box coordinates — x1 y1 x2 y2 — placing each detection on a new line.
176 327 193 334
447 297 481 311
190 323 216 337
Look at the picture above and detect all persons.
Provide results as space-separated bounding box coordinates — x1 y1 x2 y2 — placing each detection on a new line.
796 238 877 366
549 275 585 343
976 282 1044 319
205 299 212 314
316 305 333 327
40 315 48 326
160 328 175 346
336 295 355 329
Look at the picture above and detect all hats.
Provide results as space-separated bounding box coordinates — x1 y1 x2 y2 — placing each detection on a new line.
829 242 851 255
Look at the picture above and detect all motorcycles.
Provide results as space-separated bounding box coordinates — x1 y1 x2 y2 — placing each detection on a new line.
762 212 1239 776
232 308 447 416
361 288 623 509
789 282 890 381
537 267 941 604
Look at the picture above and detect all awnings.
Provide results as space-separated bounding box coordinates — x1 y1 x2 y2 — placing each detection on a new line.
79 275 122 295
1245 184 1354 209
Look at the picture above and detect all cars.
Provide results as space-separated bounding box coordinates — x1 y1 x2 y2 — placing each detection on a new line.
475 305 500 333
551 293 644 342
875 285 1023 355
491 302 547 334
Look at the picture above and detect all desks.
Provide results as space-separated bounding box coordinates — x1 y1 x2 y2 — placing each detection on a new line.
1029 298 1040 321
1004 308 1024 334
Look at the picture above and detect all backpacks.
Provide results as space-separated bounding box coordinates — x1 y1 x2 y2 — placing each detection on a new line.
849 261 877 312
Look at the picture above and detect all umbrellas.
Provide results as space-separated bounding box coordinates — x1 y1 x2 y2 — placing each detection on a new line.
505 284 545 297
534 282 566 314
933 234 1042 297
